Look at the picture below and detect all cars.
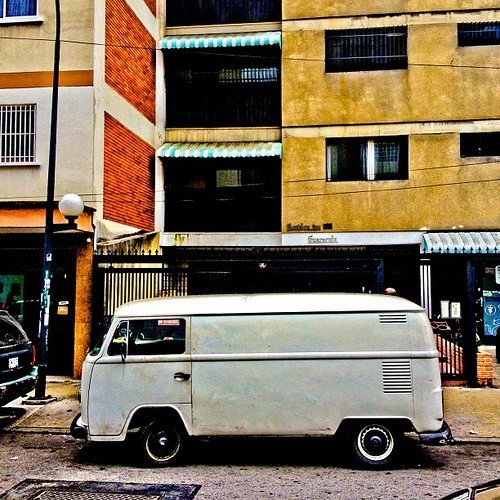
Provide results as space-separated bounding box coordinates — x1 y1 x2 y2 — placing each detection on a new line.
0 308 41 405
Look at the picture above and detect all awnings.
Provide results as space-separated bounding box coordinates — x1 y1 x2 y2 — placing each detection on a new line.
159 31 284 55
156 141 284 165
423 229 500 254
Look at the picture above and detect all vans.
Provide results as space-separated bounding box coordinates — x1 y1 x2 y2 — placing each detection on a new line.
70 292 457 468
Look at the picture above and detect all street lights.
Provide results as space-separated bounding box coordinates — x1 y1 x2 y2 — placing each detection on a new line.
24 193 84 404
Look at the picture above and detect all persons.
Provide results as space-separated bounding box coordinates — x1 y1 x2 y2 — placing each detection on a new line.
5 280 24 318
